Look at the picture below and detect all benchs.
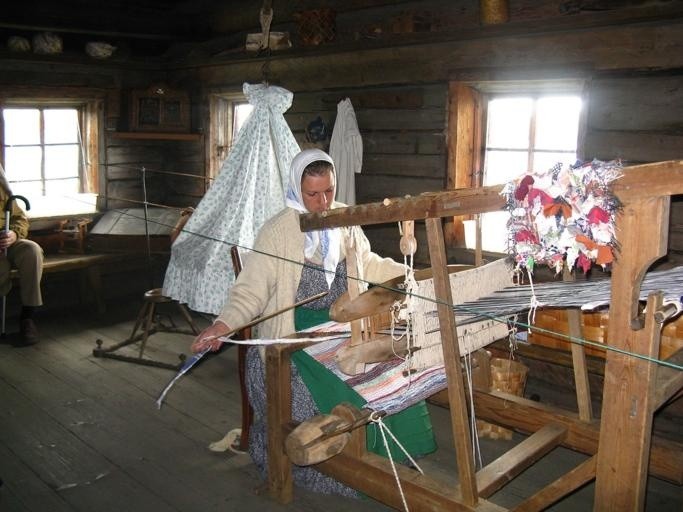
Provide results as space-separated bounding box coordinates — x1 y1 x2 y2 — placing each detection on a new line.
10 251 143 327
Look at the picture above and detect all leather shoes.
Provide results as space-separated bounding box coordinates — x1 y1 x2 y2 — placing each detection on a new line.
20 317 38 346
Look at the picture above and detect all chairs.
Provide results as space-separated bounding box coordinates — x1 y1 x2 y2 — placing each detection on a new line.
231 245 255 453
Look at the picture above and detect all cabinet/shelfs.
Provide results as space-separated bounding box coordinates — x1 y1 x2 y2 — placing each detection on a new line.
126 81 191 133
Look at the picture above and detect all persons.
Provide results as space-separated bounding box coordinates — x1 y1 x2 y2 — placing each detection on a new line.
0 162 43 348
188 146 440 503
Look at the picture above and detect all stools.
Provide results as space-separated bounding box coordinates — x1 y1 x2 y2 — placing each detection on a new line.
130 287 200 360
